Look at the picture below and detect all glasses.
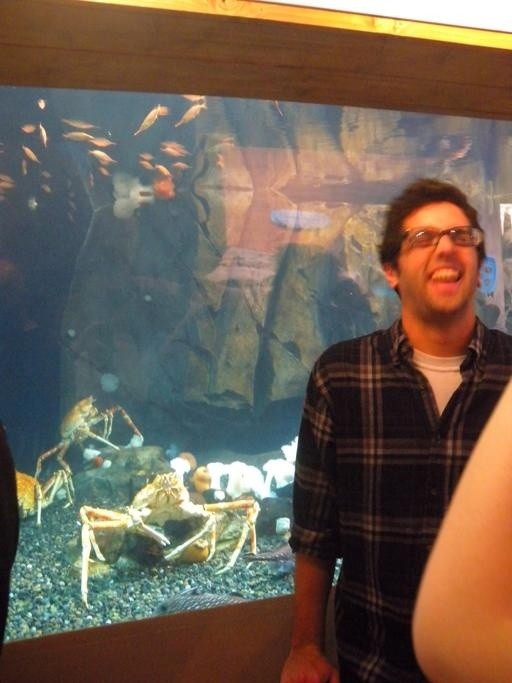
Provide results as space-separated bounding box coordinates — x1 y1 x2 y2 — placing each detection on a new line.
398 226 486 250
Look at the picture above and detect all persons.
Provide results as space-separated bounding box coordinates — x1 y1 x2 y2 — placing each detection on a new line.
279 176 512 683
0 419 19 653
411 374 512 683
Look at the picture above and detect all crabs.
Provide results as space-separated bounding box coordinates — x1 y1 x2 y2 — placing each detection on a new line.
34 393 144 478
78 471 260 609
15 467 75 526
